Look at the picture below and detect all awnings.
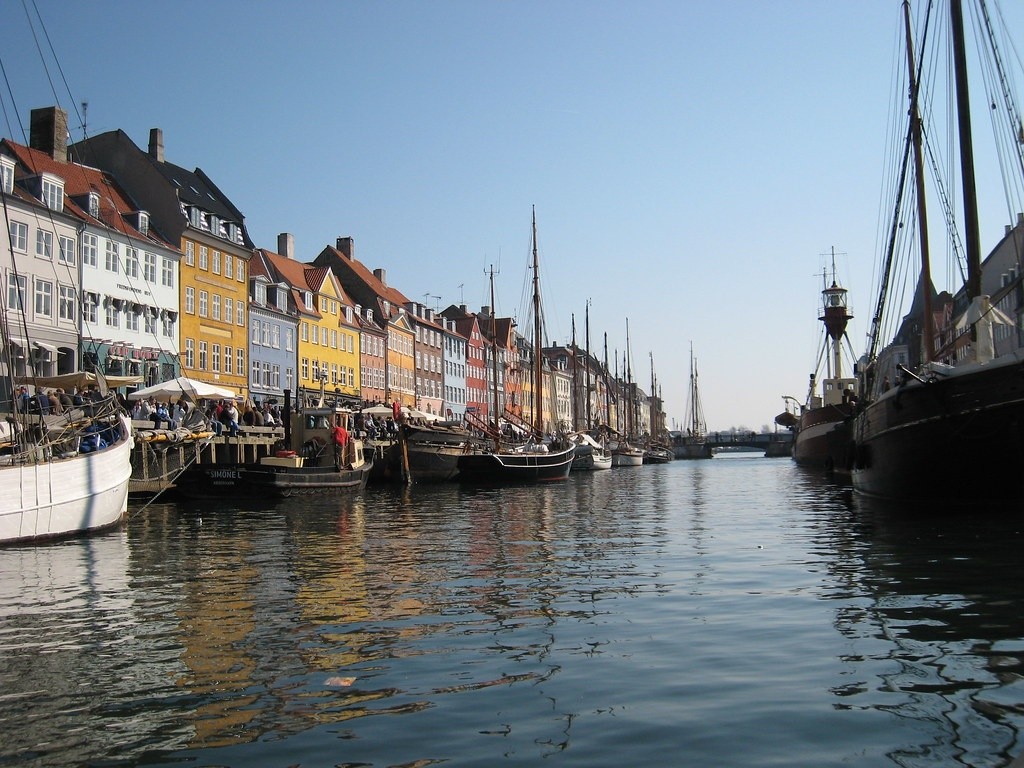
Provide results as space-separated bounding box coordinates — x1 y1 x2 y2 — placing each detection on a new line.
10 338 66 354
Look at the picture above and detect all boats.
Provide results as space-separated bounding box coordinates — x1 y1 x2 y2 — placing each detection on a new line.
233 378 377 501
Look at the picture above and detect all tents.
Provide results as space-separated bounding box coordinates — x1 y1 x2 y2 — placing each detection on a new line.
16 373 145 385
419 411 446 421
128 377 234 402
400 406 426 422
354 405 394 416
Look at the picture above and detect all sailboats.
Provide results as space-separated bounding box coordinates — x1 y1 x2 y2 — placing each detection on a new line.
762 0 1024 510
383 203 713 486
1 0 218 545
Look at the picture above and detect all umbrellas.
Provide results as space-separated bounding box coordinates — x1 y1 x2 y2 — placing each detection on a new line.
955 296 1014 328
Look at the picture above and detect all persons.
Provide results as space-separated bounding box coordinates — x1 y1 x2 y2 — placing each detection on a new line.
881 375 899 392
12 385 524 444
715 431 778 443
333 426 350 472
